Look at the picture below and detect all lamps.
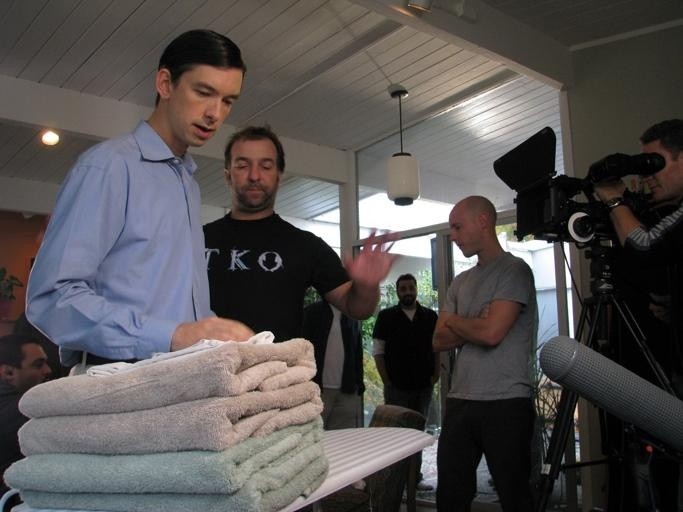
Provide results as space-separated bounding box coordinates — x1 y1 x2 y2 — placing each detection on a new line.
386 90 419 205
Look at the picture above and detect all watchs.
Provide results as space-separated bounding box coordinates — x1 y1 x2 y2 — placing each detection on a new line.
605 196 627 215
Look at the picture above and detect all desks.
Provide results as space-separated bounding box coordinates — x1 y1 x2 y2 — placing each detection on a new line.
0 429 435 512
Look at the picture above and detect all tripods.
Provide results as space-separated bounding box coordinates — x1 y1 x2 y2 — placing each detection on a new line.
540 247 683 512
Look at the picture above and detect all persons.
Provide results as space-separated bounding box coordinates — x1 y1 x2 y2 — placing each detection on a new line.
592 116 683 512
370 272 438 493
0 335 52 485
301 286 371 489
21 28 255 367
196 122 394 350
429 194 549 511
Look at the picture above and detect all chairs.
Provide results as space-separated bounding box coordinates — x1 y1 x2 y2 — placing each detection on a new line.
320 405 426 512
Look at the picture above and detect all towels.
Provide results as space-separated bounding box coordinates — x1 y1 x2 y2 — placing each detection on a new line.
3 337 329 512
